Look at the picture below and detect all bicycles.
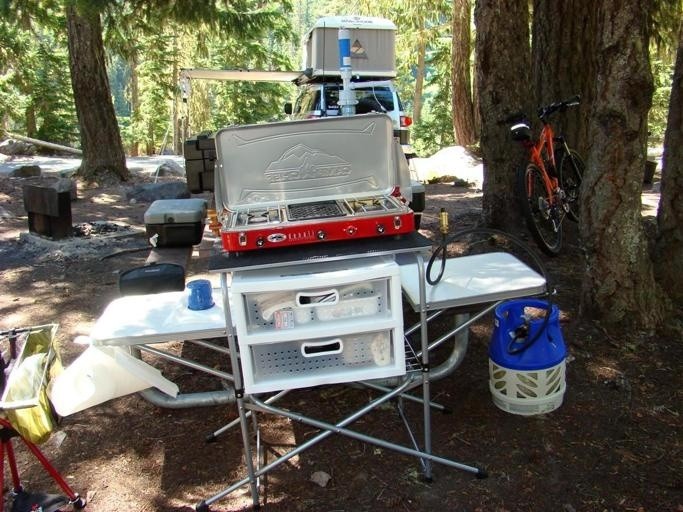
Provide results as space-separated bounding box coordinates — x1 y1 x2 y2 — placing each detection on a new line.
495 94 588 256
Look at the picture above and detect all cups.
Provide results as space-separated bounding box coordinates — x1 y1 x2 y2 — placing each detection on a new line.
186 281 215 310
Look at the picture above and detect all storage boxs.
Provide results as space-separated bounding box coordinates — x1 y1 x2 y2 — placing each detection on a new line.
218 118 415 258
145 200 207 247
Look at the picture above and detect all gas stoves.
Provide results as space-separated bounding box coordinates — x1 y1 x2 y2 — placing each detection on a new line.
219 193 413 252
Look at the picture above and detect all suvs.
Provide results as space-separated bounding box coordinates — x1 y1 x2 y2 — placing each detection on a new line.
284 81 411 168
